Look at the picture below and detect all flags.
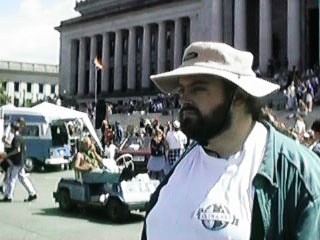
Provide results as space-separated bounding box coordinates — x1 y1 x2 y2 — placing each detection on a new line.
93 56 103 69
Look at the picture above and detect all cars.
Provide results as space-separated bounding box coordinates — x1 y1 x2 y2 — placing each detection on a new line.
118 136 152 173
53 154 160 223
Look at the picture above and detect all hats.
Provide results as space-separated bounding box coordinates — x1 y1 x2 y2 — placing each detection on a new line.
173 120 181 128
149 41 280 98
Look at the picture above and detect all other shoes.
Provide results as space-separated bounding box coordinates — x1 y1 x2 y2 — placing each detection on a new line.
24 195 37 202
0 199 11 202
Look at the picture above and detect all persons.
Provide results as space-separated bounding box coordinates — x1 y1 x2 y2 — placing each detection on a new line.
0 59 320 202
141 41 320 240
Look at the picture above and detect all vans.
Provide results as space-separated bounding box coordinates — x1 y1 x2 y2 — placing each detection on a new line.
1 106 77 173
